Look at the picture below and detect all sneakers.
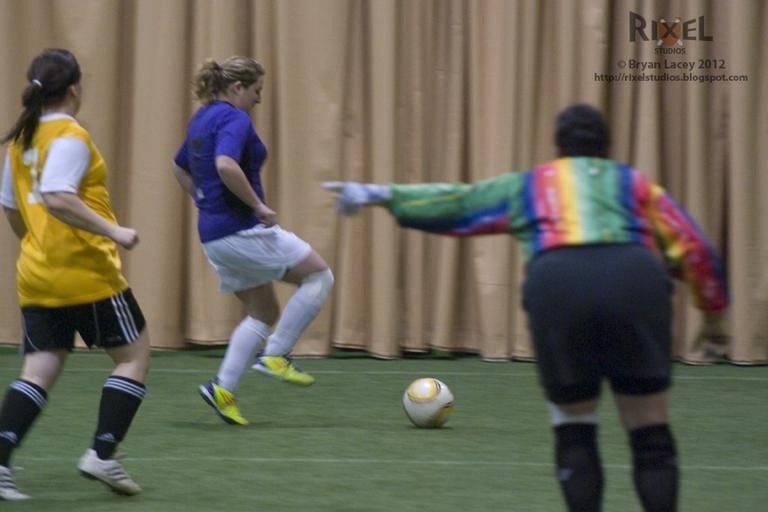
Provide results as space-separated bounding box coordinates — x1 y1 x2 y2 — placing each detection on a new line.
77 449 140 493
200 377 248 426
251 352 315 387
1 467 27 499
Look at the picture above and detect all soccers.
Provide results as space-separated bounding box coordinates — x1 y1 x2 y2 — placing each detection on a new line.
403 377 455 427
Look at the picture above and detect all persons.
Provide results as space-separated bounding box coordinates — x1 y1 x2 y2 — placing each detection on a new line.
318 104 734 512
167 55 334 426
1 47 151 502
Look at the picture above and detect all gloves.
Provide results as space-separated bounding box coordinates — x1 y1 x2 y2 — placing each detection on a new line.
688 318 728 358
324 181 389 217
